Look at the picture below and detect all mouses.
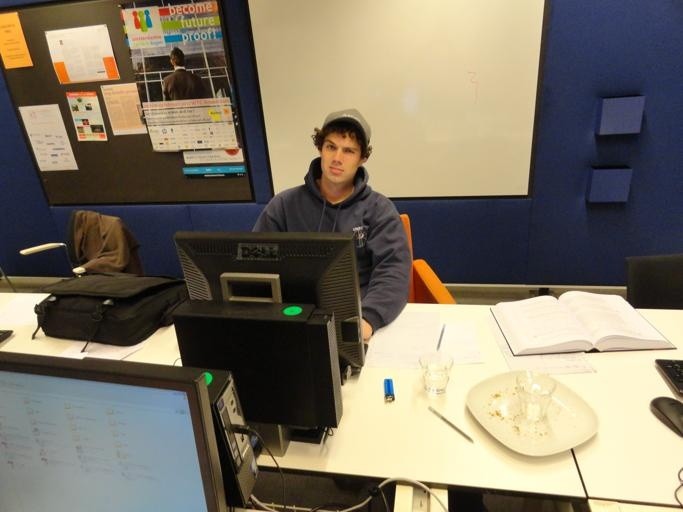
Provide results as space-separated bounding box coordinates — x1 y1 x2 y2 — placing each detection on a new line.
650 396 683 434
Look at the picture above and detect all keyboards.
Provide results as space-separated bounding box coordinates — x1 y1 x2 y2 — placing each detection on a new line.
656 358 683 394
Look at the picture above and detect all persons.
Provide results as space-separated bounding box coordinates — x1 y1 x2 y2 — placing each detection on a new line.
253 109 412 341
162 46 205 99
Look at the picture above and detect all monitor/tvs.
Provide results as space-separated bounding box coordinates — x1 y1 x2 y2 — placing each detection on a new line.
181 231 366 369
0 350 224 512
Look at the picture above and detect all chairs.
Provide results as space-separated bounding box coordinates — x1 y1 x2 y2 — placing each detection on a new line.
17 208 145 277
394 212 459 306
622 252 682 311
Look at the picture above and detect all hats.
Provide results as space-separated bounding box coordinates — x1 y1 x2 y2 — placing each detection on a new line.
322 109 372 142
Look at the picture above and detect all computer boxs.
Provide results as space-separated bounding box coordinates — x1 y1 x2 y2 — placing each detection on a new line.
170 298 344 428
84 354 261 508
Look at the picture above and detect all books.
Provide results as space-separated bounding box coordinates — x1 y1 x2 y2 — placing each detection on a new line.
488 291 677 356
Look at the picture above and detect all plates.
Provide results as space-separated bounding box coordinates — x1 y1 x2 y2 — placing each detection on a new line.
465 372 599 458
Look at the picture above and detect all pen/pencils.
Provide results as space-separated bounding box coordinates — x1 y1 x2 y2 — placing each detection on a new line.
428 406 474 443
436 324 446 352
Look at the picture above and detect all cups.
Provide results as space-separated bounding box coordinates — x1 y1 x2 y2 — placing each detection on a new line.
511 371 556 436
417 351 454 394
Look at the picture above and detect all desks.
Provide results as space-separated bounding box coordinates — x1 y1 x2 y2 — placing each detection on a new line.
542 298 683 510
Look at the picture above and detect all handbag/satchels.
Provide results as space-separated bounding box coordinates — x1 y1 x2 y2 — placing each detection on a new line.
34 270 191 346
